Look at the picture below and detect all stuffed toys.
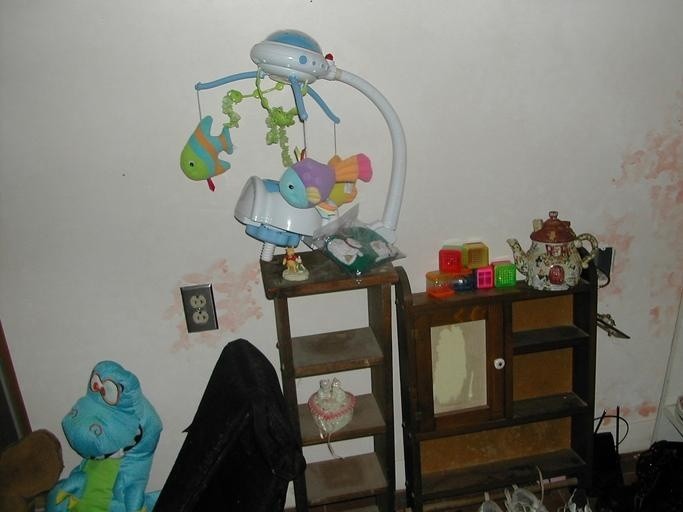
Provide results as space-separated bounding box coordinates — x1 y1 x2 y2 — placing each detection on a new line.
47 360 164 511
178 29 406 257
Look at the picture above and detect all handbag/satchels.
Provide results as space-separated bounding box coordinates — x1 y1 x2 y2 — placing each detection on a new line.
151 337 307 512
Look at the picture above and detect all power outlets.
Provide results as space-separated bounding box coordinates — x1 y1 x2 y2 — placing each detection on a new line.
175 285 221 334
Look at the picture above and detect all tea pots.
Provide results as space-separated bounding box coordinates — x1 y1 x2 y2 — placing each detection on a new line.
506 210 600 293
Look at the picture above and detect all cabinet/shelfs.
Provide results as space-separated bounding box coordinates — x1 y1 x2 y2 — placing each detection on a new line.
258 255 398 511
392 244 597 511
